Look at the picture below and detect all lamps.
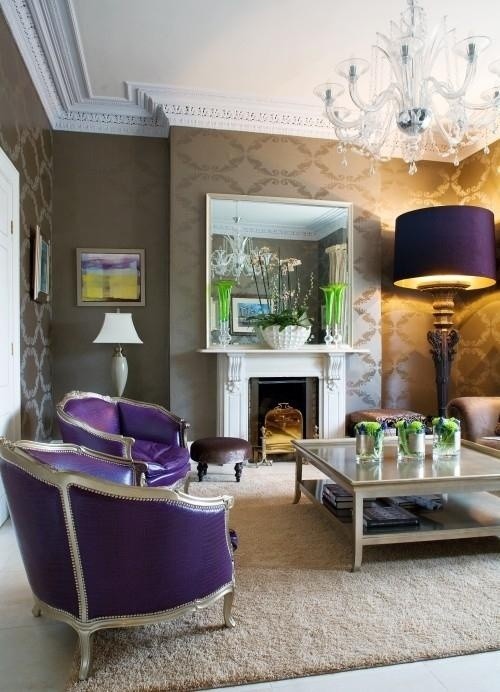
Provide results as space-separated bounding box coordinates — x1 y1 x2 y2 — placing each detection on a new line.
393 206 496 414
90 308 143 397
314 0 500 176
210 217 272 285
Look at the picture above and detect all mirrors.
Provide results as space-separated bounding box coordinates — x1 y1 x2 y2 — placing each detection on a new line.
206 193 353 348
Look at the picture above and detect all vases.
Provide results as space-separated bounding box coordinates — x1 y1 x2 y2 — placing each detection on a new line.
260 322 312 348
331 283 349 344
319 284 334 344
218 280 227 344
222 279 231 344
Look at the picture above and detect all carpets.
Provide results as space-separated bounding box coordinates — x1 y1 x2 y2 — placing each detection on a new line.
66 463 500 692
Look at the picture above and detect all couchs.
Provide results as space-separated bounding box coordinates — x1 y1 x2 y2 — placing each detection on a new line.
446 397 500 459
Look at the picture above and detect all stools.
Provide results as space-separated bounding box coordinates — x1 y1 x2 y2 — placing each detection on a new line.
350 408 428 437
190 437 253 482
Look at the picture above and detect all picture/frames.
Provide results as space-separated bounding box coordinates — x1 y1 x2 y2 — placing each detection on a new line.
76 247 146 307
229 293 278 336
34 224 51 304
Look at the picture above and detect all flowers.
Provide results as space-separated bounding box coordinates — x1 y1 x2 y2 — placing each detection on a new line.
250 246 315 332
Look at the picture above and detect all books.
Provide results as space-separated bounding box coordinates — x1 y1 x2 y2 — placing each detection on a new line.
323 484 374 501
322 492 370 509
354 508 420 526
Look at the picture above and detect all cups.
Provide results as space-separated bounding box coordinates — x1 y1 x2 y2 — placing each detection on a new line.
354 416 462 468
